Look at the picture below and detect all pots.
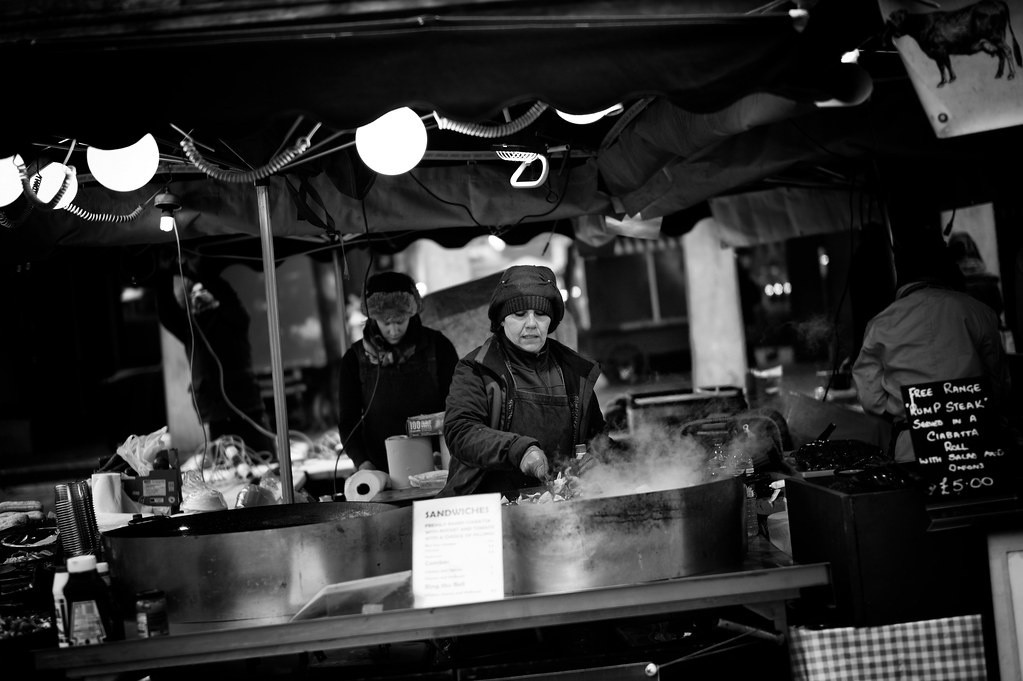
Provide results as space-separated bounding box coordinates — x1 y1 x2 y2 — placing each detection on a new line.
100 501 398 624
499 457 746 597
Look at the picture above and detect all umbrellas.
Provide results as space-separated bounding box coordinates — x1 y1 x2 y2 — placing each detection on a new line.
0 1 877 505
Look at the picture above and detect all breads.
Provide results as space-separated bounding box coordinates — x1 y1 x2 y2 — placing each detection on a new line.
0 500 46 530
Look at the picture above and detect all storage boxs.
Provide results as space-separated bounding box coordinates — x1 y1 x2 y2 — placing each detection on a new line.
785 462 923 608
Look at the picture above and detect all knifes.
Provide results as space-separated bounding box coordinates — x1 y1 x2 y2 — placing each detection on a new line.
543 474 555 496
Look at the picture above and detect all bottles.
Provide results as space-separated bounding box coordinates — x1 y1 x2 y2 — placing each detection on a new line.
62 555 119 647
574 444 587 460
724 443 761 556
52 572 69 648
96 562 126 641
135 588 170 637
706 440 729 474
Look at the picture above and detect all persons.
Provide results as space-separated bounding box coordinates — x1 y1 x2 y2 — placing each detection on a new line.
435 266 628 498
852 240 1011 462
949 233 985 274
154 258 273 448
337 271 458 472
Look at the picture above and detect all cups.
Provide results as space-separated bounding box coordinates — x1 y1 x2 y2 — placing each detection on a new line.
385 435 442 490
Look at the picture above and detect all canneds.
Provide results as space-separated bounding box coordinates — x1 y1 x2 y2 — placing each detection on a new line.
135 589 169 638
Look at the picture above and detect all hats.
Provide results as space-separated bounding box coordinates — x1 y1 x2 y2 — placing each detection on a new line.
487 265 564 336
359 272 423 320
